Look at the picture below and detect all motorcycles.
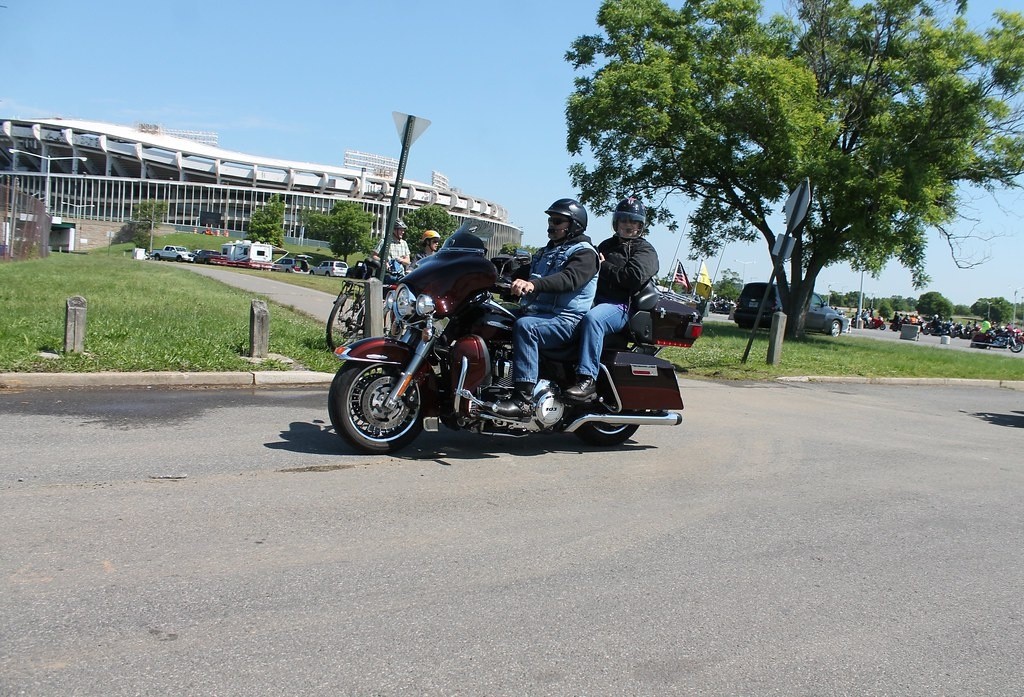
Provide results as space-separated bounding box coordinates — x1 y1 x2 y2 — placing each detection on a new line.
710 300 736 314
327 219 705 454
850 315 1024 353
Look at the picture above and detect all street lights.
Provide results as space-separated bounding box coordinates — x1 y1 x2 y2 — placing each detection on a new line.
986 301 993 317
62 201 96 219
8 148 88 213
858 267 866 316
827 285 831 306
734 259 756 289
1009 285 1024 328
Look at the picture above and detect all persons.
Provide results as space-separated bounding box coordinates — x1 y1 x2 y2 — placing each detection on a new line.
931 314 1024 349
893 311 921 332
713 296 737 314
855 306 875 329
484 198 601 423
411 230 441 267
831 306 838 313
371 218 411 333
563 197 659 402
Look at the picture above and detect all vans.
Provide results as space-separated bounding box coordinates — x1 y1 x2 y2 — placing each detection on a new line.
733 282 849 338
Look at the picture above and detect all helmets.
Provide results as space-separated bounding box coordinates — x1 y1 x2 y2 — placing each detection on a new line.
544 198 587 239
394 220 408 229
613 198 646 238
422 230 441 242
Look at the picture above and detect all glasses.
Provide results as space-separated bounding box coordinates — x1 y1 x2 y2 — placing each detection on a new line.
547 217 569 225
430 239 439 244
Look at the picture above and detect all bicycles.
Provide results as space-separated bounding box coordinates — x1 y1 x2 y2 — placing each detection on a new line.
326 268 405 350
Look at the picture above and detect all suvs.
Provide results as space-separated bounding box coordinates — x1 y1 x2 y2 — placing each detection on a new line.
310 261 349 277
346 260 365 279
273 254 314 275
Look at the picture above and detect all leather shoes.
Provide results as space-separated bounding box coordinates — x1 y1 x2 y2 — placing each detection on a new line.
566 377 597 402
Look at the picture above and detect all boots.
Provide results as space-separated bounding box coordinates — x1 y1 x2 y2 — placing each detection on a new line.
483 381 535 422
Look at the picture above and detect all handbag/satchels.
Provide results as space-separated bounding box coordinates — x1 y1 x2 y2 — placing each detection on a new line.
384 260 405 284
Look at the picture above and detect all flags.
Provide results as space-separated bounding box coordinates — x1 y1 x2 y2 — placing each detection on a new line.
673 260 693 294
697 263 713 299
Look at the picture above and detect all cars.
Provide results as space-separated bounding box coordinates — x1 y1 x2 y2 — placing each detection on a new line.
192 249 201 255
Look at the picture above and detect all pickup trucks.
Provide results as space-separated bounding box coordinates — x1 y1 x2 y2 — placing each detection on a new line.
194 250 228 267
151 245 196 263
